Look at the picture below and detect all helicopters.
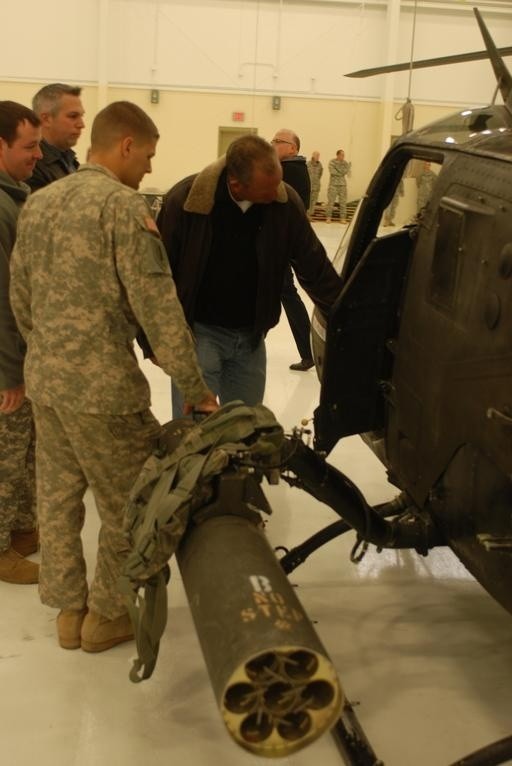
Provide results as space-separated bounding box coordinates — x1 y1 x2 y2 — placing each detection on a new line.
158 1 511 764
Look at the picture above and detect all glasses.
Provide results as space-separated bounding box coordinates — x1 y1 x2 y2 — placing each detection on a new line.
270 139 293 145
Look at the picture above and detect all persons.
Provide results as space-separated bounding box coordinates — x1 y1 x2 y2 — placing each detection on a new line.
1 100 44 586
137 134 347 427
304 150 323 222
266 128 316 371
21 83 85 195
325 150 349 224
382 180 404 228
415 160 438 215
8 101 220 654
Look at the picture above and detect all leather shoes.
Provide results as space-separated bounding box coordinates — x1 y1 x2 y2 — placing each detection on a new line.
289 358 315 371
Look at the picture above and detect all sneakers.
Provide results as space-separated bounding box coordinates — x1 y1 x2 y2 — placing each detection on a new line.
0 526 41 585
54 606 137 654
383 219 394 227
326 217 332 224
340 218 347 224
